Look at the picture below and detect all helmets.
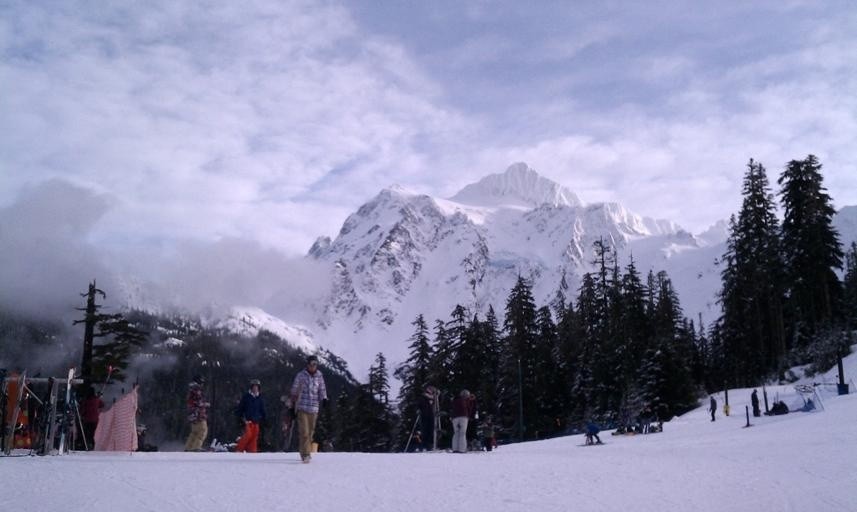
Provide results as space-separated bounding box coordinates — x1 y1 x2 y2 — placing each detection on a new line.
249 379 261 391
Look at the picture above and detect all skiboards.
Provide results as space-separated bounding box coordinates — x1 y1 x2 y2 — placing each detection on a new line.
577 443 608 446
59 368 78 455
36 377 58 456
0 368 27 455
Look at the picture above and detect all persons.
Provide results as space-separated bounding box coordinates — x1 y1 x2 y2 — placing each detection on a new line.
233 378 267 453
446 388 476 452
287 354 331 465
793 397 815 412
73 385 105 452
583 419 602 445
763 400 789 416
182 381 212 453
413 383 441 451
707 395 719 423
749 387 761 418
